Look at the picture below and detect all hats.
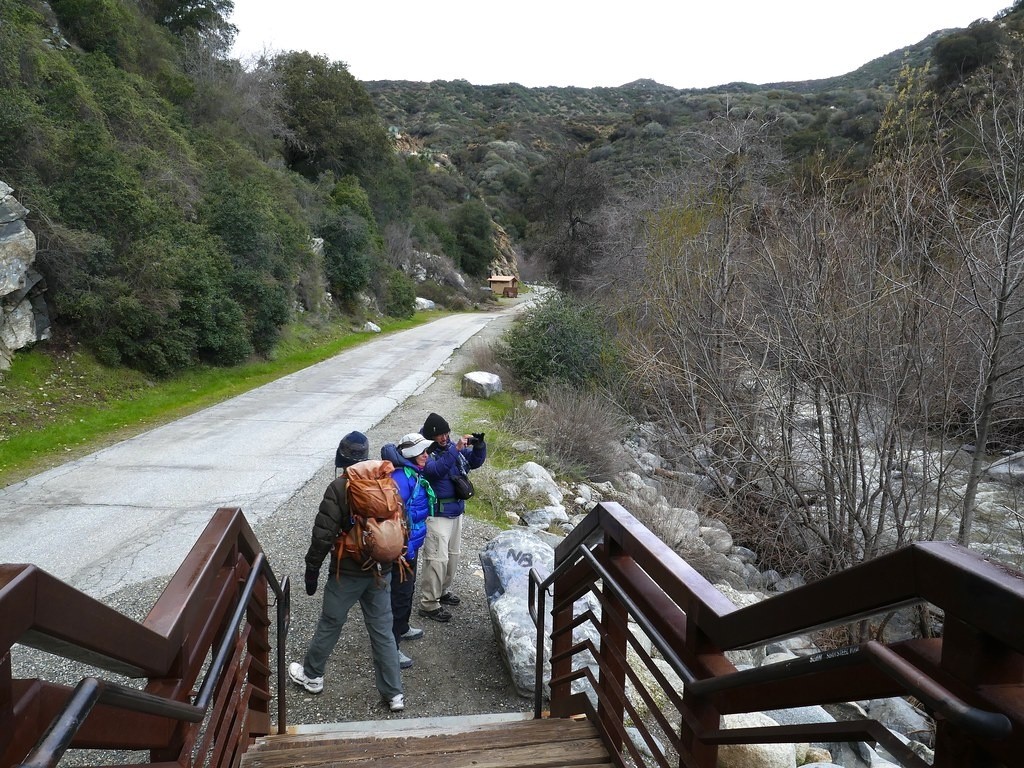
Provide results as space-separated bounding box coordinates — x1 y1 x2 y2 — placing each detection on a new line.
335 431 369 468
423 413 450 436
398 433 434 458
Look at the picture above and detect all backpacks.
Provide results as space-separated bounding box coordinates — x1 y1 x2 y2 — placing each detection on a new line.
334 460 414 583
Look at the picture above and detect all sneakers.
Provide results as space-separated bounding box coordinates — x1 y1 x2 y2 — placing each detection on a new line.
288 662 324 693
389 693 405 711
440 592 460 604
401 625 423 640
398 650 414 667
418 606 452 622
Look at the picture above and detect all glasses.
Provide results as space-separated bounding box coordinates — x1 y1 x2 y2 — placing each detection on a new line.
420 449 428 455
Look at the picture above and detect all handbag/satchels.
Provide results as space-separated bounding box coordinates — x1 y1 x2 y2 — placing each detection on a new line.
450 475 474 500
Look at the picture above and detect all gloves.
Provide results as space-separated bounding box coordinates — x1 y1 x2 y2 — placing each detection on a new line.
305 570 319 596
472 432 485 443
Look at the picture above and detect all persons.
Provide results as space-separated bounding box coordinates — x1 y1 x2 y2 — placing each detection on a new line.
287 431 405 711
419 412 487 621
381 433 434 667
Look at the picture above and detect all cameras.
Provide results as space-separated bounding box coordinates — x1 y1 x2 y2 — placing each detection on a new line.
465 435 479 446
